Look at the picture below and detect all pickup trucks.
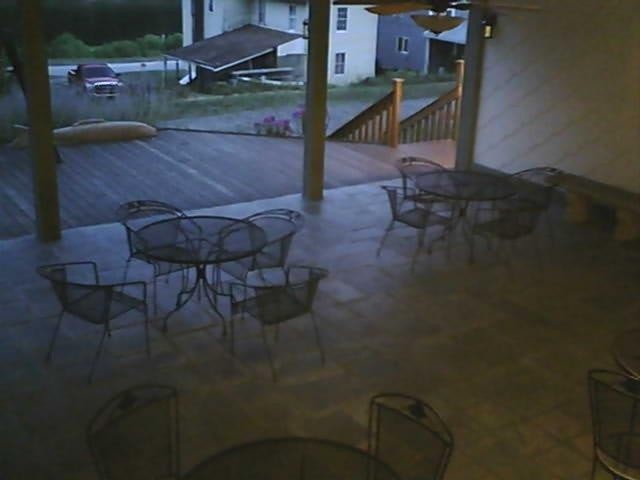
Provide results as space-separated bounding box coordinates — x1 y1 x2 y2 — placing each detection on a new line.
67 61 127 101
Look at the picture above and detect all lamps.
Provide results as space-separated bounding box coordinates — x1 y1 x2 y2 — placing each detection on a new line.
481 13 497 39
410 6 465 35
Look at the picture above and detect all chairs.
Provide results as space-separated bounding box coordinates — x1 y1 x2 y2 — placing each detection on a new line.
579 365 639 478
462 163 563 270
36 259 153 387
84 387 178 479
226 263 331 385
114 201 206 316
375 182 476 275
211 208 306 322
395 156 466 226
366 392 457 479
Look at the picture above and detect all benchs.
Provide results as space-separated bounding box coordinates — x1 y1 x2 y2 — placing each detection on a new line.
542 170 639 244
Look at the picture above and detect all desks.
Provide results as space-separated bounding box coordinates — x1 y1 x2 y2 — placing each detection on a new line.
612 323 639 380
130 213 270 337
181 435 401 479
410 167 519 267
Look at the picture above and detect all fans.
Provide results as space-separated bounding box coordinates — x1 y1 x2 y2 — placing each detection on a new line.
332 1 543 16
